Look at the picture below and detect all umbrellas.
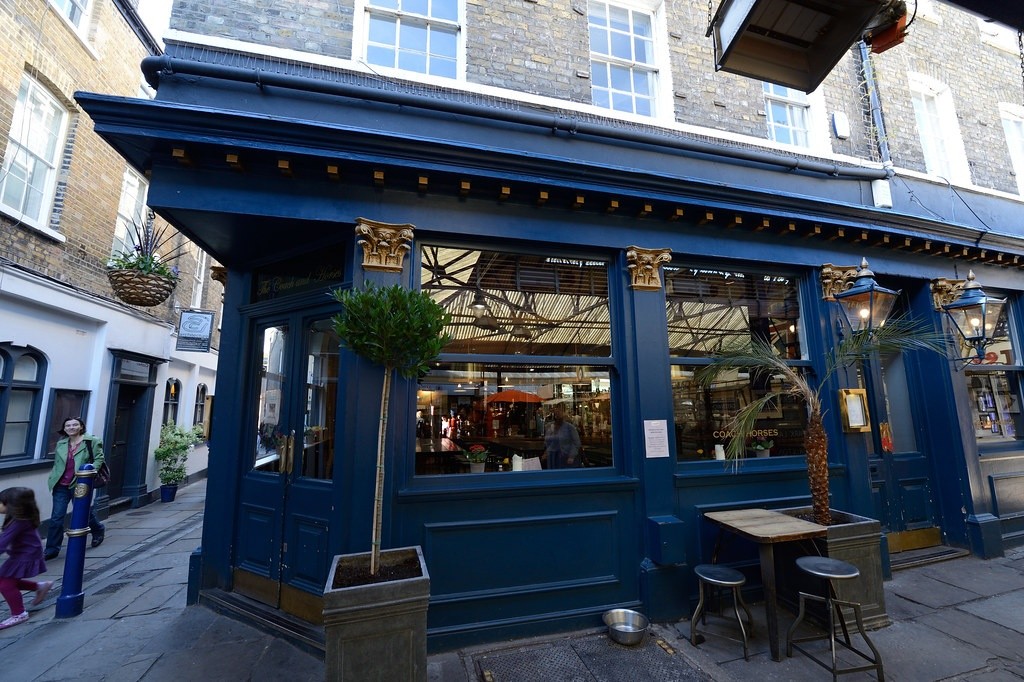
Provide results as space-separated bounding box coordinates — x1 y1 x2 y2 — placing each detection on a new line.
483 389 543 403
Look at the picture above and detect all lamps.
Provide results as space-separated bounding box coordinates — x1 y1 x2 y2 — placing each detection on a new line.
833 257 902 367
943 270 1008 361
513 326 531 338
470 316 499 333
467 291 490 321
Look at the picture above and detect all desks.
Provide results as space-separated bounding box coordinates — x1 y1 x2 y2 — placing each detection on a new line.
491 438 546 471
700 508 829 661
416 437 464 473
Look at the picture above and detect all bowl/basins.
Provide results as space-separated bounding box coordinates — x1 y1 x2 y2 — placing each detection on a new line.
603 609 649 645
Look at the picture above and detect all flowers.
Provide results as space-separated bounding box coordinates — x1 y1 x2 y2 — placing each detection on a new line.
101 213 190 280
302 425 326 437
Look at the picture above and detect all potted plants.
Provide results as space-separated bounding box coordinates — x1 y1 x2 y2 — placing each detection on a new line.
322 278 453 681
150 419 205 503
675 311 953 636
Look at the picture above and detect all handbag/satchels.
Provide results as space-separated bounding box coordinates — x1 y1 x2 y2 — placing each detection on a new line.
568 424 588 468
86 434 111 488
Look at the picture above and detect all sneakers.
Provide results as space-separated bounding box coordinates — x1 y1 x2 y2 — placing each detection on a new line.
0 612 29 628
35 579 53 604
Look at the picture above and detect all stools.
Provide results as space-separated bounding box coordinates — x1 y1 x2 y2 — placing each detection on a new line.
688 564 755 662
787 555 886 682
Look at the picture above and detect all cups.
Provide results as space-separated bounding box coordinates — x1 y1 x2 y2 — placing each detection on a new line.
992 424 998 432
980 416 992 428
989 413 996 420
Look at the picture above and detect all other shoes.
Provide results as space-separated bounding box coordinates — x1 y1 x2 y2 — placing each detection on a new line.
44 552 57 559
90 527 104 548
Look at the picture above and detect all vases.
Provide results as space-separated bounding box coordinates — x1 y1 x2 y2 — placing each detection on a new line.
307 435 315 445
108 267 178 309
313 434 321 441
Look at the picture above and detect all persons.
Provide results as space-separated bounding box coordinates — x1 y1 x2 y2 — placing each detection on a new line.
416 409 429 438
45 415 106 562
441 414 450 436
544 401 589 468
0 486 52 631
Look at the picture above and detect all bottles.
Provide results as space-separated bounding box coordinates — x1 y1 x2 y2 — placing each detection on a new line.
977 395 986 412
451 415 455 427
487 405 544 438
499 465 503 472
